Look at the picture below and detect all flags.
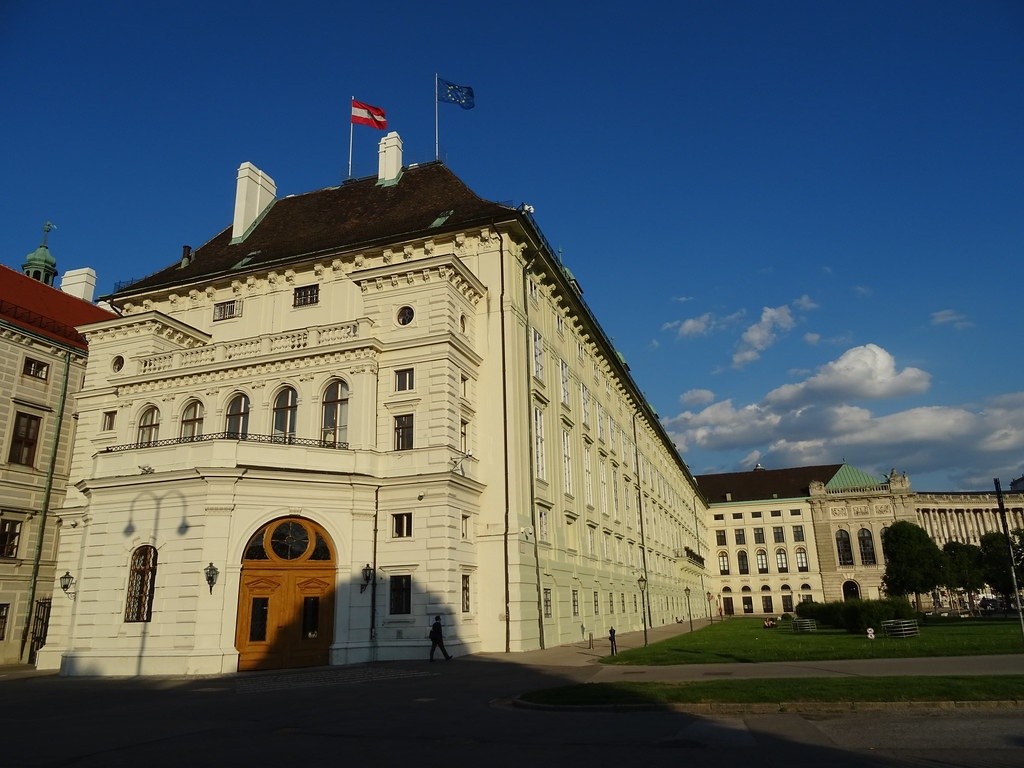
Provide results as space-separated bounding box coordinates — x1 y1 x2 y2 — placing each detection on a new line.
351 96 387 130
436 73 474 110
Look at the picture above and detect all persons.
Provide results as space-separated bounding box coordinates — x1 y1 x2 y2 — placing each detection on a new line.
912 598 916 609
429 616 453 662
889 468 898 485
719 607 723 616
949 597 969 610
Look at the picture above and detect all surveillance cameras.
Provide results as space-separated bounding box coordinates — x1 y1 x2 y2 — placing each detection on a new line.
469 451 473 458
71 521 77 527
419 494 424 499
529 529 533 535
138 464 149 469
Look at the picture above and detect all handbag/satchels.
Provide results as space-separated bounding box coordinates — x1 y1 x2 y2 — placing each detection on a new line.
429 630 434 639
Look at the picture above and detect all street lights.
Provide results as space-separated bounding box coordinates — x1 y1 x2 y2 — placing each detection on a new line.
706 591 713 624
717 593 723 621
684 586 693 631
636 574 650 646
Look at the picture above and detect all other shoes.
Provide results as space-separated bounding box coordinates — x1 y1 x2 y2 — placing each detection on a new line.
429 659 435 662
446 655 452 662
